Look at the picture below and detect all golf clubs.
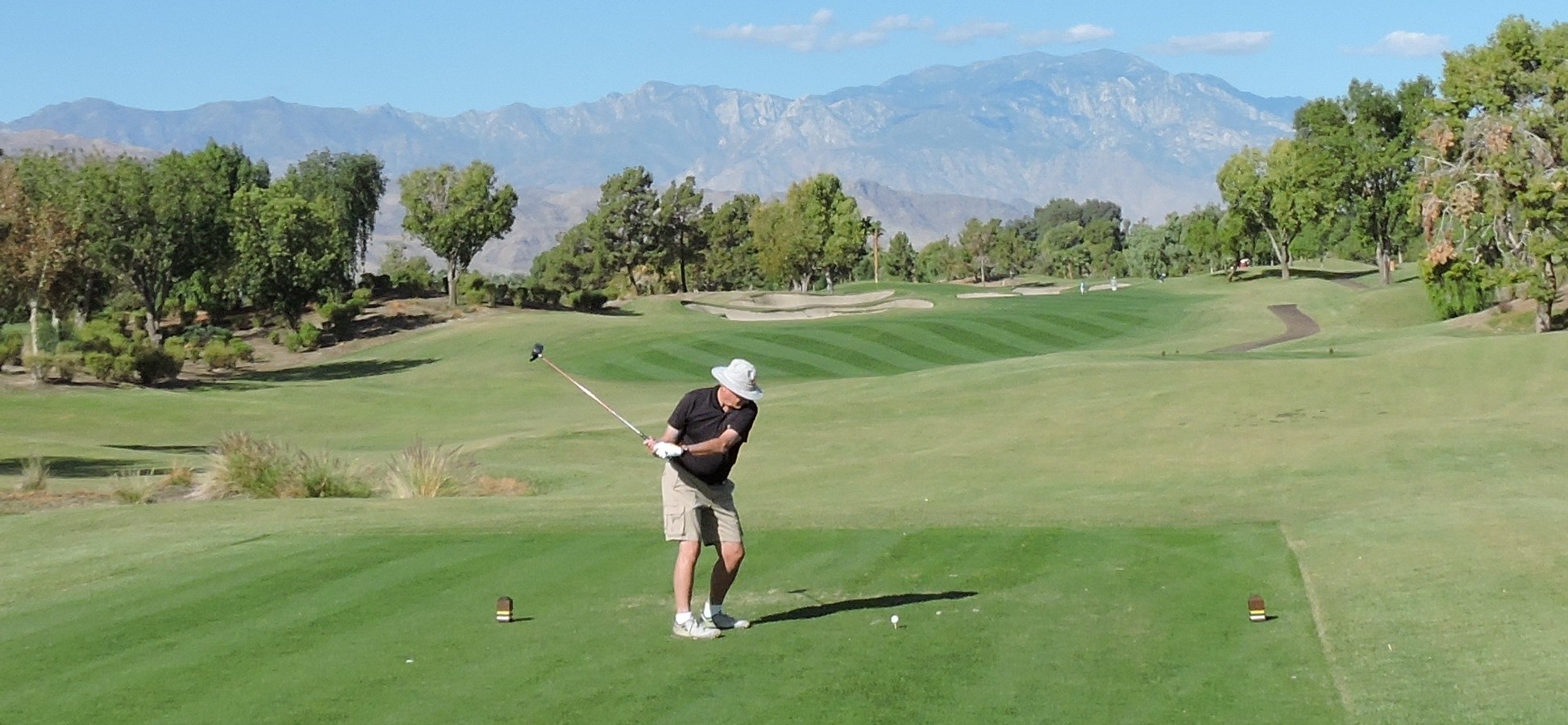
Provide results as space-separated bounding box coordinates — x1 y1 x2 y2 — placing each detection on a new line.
532 343 647 442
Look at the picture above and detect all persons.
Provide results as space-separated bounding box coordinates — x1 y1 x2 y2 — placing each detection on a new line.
643 357 764 639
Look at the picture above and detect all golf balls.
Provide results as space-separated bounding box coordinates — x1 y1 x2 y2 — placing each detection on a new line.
891 616 899 623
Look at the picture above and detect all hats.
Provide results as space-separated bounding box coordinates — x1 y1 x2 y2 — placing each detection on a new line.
711 359 763 400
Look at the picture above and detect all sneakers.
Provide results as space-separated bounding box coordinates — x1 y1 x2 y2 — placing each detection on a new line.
699 609 750 628
671 616 721 639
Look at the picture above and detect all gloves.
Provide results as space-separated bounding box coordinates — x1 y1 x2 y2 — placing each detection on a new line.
654 441 682 460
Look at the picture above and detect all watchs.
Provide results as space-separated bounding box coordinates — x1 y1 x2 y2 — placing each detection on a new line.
679 444 689 457
